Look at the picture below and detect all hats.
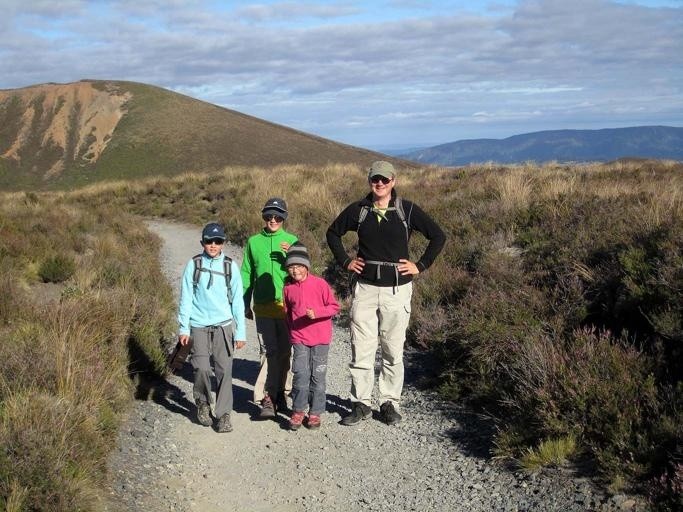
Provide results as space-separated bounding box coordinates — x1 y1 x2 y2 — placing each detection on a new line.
369 160 396 181
284 241 311 272
261 198 288 221
202 222 226 242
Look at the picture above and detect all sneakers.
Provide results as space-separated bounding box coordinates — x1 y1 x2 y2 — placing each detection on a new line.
212 413 234 433
306 413 321 430
286 411 306 430
260 394 276 419
195 398 214 426
340 401 373 427
372 400 403 426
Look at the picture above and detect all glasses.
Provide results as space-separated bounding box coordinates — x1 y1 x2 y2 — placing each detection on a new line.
203 238 224 245
287 265 305 270
262 214 283 223
368 176 393 184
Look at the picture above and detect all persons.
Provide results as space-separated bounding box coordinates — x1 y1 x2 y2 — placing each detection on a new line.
176 222 247 432
280 239 343 428
242 196 299 417
326 158 447 426
